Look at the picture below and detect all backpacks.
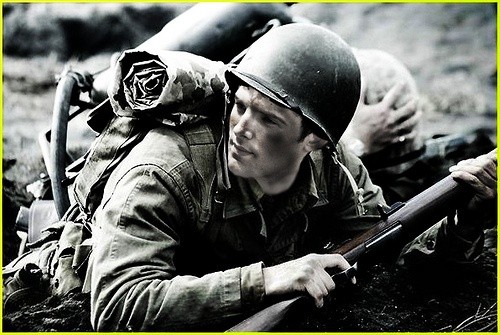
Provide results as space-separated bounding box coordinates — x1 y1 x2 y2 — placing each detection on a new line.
70 48 222 230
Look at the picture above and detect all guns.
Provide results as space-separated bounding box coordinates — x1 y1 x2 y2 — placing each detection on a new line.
224 148 497 332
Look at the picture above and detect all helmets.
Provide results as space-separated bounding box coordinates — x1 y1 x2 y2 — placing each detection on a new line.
224 21 362 152
358 47 425 160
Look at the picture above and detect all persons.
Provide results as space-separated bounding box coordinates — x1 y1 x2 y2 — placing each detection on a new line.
2 3 498 332
